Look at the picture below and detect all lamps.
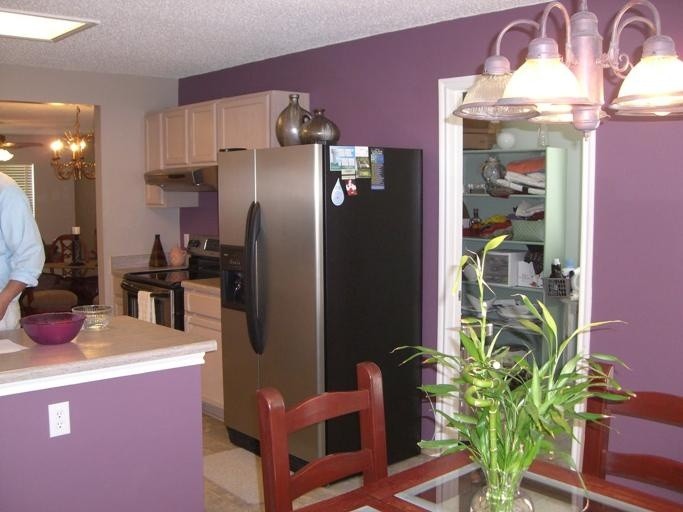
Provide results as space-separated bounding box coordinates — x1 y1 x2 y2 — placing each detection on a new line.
50 107 96 181
451 0 682 132
69 227 84 266
0 135 14 162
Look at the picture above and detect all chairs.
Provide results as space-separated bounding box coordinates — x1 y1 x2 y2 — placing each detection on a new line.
48 233 88 263
581 364 683 494
256 362 389 512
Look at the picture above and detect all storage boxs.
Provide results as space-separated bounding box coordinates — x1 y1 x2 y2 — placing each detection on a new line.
463 128 495 150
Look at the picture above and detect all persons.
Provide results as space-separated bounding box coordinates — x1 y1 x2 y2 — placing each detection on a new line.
0 172 45 332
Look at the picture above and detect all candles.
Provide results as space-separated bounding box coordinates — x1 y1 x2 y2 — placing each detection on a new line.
58 150 60 157
53 150 56 157
73 151 75 159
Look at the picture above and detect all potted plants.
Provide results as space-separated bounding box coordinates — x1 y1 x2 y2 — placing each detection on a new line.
388 235 636 511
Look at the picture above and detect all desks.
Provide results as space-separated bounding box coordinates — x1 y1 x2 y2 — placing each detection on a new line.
0 315 218 511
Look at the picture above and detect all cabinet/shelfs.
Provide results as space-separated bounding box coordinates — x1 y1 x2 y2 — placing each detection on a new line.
143 90 310 208
181 276 221 423
462 146 566 386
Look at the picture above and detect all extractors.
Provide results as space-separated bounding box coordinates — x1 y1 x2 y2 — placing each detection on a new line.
144 165 218 192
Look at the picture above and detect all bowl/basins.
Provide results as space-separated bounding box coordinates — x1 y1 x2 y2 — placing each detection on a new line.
72 305 113 332
19 313 86 345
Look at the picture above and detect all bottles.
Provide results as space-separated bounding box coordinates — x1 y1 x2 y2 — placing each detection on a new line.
149 234 168 280
548 258 567 297
470 208 482 237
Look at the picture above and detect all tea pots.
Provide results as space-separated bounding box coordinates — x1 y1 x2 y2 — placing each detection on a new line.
168 245 187 267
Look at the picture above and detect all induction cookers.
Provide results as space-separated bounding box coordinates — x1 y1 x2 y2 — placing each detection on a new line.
187 235 219 258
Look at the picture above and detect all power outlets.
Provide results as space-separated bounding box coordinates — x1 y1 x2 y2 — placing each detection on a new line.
47 400 71 438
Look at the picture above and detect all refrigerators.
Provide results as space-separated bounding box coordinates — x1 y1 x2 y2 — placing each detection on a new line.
216 143 422 488
